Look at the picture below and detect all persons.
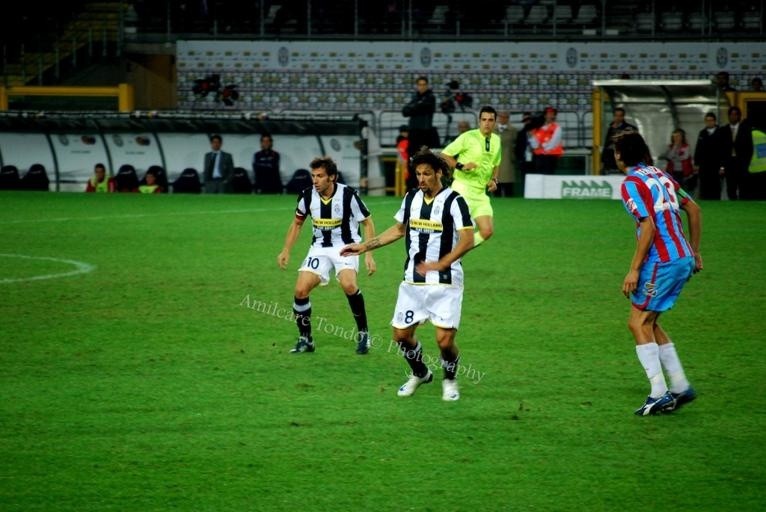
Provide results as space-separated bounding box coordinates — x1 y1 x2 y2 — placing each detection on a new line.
752 79 762 91
137 172 161 194
278 158 376 354
253 134 283 195
203 135 233 193
339 153 474 403
695 113 723 200
714 72 736 91
396 76 563 266
86 164 117 193
718 106 753 202
666 128 694 194
613 133 705 416
740 119 766 199
600 108 638 175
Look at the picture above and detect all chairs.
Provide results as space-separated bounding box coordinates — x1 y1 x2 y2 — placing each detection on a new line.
0 164 312 194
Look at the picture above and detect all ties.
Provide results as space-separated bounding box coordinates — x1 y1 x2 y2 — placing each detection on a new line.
212 153 216 167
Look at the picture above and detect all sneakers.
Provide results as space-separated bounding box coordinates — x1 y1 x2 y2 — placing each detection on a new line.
355 331 370 354
397 369 433 397
289 336 315 354
441 379 459 401
671 385 696 407
634 390 676 417
